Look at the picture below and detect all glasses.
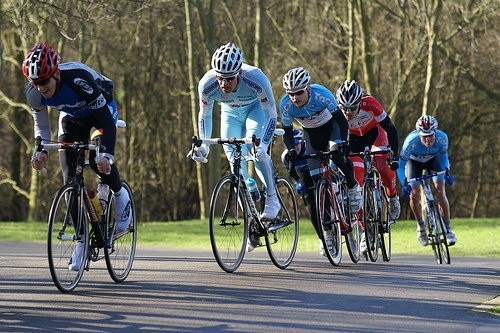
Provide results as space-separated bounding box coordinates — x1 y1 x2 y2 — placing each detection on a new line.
215 73 239 80
287 86 307 96
341 105 358 110
29 76 53 85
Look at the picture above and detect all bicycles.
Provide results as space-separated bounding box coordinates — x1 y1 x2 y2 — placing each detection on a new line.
31 120 140 293
403 167 456 264
286 140 363 266
346 146 401 263
192 126 299 273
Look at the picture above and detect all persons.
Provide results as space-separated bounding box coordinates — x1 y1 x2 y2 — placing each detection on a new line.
397 116 458 246
278 67 401 257
187 42 282 251
21 42 132 272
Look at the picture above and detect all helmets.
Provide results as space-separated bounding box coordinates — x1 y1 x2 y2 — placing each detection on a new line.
22 43 60 82
336 79 362 106
212 43 243 78
416 115 438 136
283 67 311 91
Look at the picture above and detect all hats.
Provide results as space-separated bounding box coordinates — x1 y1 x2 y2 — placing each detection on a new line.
283 129 304 145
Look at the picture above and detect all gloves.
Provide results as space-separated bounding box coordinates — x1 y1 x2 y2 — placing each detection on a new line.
296 183 304 194
187 144 209 163
285 149 298 162
444 174 455 185
402 185 412 195
386 153 399 170
251 143 268 162
338 142 350 156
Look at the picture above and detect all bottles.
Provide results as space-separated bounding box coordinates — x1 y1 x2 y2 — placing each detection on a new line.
246 177 261 203
96 184 109 216
374 188 381 208
88 191 103 217
332 182 342 203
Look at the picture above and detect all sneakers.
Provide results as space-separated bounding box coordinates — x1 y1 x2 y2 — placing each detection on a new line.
387 196 401 219
320 231 336 257
445 232 457 244
360 232 372 252
247 237 260 252
112 187 132 232
261 197 281 220
417 224 427 245
70 242 83 270
347 179 363 213
